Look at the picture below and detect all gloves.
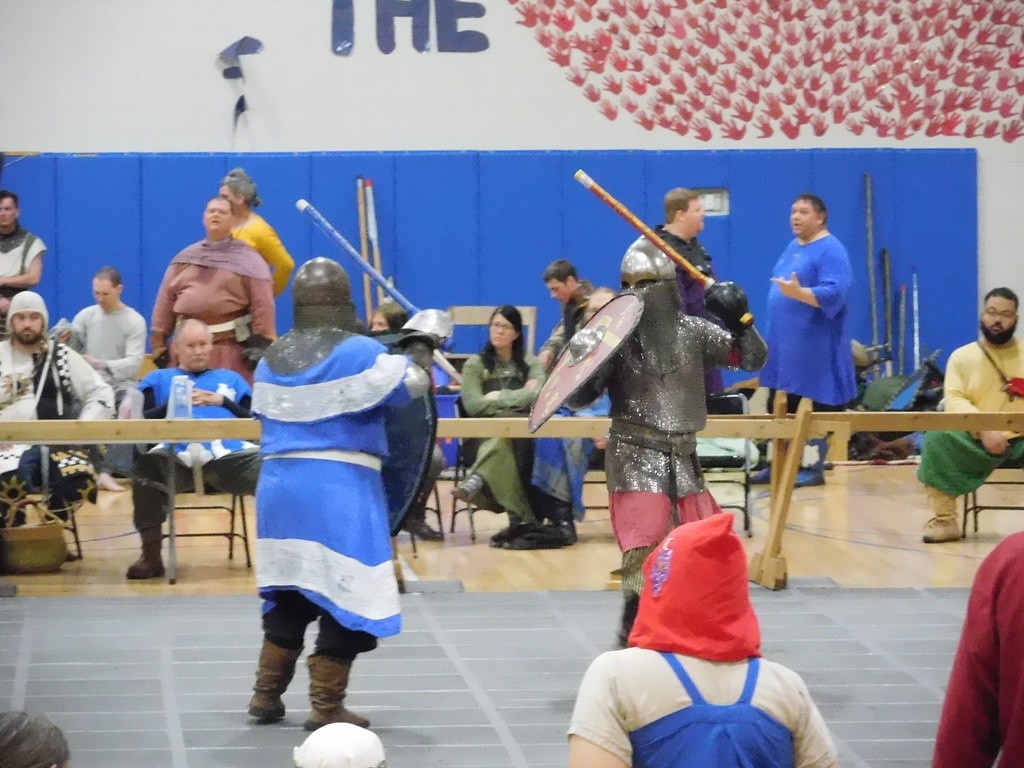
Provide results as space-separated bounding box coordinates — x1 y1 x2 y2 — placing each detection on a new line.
706 283 751 337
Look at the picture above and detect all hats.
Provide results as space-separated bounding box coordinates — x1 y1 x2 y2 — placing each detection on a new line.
6 291 48 335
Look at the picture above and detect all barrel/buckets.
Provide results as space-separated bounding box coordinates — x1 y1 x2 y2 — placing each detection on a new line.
2 498 67 572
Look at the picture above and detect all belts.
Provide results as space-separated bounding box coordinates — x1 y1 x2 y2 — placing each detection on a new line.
610 421 695 446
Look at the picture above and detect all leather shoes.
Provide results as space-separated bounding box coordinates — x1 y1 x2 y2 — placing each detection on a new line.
404 523 444 540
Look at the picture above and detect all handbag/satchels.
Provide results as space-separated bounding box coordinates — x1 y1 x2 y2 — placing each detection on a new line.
0 500 68 573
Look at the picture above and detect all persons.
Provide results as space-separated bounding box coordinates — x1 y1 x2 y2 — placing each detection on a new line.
747 194 858 487
120 319 261 581
0 291 115 563
513 258 612 548
0 711 71 768
565 511 840 768
53 268 147 493
150 165 296 386
247 256 454 732
291 720 388 768
931 533 1024 768
0 191 47 340
371 300 409 332
450 305 546 548
655 187 724 395
916 287 1023 543
527 231 769 647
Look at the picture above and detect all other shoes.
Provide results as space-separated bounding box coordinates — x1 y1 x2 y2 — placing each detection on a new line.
556 520 577 544
453 474 485 501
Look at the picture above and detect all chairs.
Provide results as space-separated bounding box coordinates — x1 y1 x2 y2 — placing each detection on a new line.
162 488 253 566
962 465 1024 539
451 396 483 540
697 393 754 535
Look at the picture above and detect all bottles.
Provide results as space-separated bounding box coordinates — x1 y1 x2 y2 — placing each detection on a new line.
172 375 194 419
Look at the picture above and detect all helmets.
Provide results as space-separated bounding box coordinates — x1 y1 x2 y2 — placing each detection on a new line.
293 722 385 768
621 234 675 287
292 257 350 305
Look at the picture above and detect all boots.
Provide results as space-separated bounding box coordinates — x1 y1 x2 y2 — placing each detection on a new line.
923 486 962 543
305 655 370 730
127 506 166 578
248 639 305 716
795 439 827 486
751 468 769 484
